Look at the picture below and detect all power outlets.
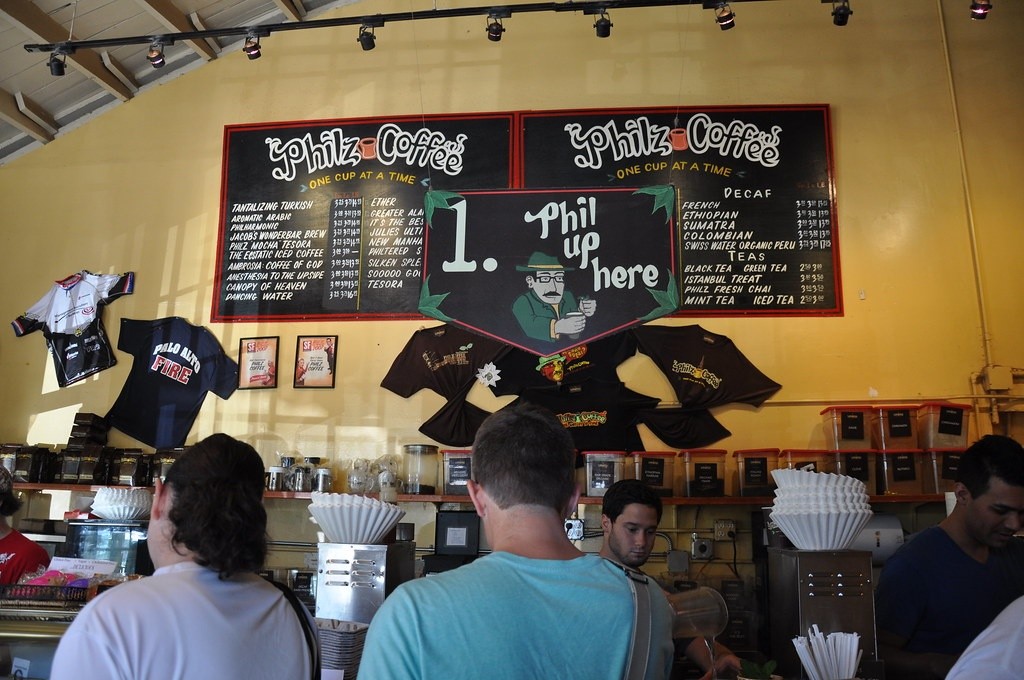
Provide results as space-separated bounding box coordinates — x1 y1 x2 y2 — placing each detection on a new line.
714 518 735 542
692 538 713 561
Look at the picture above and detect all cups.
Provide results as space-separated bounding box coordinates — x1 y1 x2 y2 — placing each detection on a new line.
264 444 404 495
665 586 727 641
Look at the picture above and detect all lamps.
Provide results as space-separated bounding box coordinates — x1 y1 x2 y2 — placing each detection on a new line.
592 4 614 40
147 38 167 69
823 1 852 28
47 50 68 76
242 33 263 60
354 24 377 51
970 1 992 19
487 13 506 43
705 2 736 30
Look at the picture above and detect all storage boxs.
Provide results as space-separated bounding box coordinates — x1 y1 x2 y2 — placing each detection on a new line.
67 412 110 449
632 451 677 497
441 449 472 498
779 402 976 500
684 448 727 499
582 450 625 500
734 447 779 493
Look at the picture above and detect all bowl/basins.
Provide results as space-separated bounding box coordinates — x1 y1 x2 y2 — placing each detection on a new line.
769 468 874 552
306 492 407 545
91 487 153 521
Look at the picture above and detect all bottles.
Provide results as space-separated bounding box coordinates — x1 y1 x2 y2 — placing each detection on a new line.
403 443 439 495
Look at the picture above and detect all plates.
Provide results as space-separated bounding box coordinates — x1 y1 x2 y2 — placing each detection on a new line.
312 617 369 680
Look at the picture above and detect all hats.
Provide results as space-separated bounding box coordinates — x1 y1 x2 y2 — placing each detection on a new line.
0 466 13 492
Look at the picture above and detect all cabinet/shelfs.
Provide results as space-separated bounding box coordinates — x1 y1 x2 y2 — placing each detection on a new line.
9 485 155 546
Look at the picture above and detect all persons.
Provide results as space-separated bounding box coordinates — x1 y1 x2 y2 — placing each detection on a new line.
356 405 676 680
589 479 783 680
48 434 321 680
944 594 1024 680
875 434 1024 680
0 462 51 601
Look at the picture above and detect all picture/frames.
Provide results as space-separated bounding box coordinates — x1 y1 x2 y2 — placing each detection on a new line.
237 337 279 388
292 335 338 388
